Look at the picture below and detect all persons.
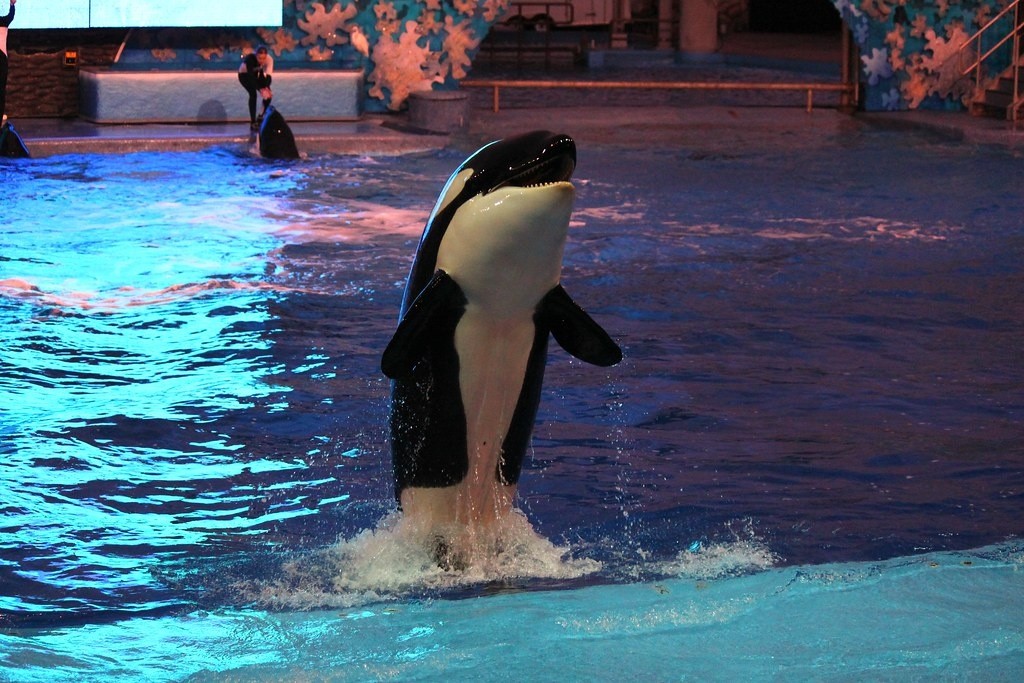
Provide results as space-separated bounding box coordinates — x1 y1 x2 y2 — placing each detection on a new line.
238 47 274 131
0 0 16 120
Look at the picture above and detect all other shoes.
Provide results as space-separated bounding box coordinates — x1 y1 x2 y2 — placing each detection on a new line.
250 121 255 131
257 117 263 125
